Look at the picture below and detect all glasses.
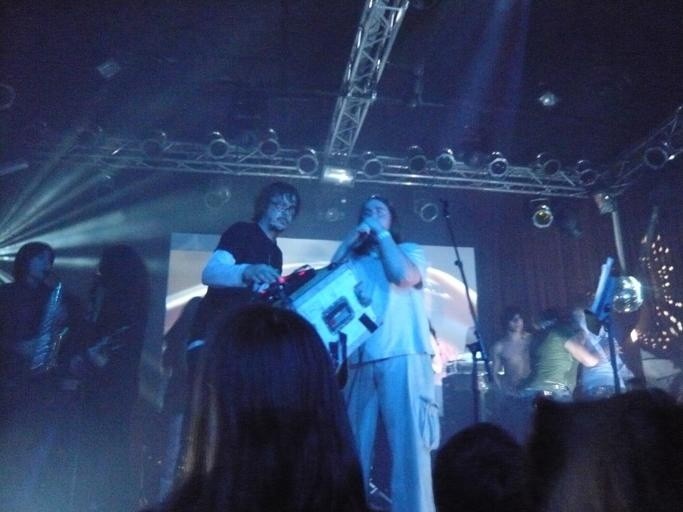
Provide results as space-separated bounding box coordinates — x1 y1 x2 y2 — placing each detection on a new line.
272 201 296 217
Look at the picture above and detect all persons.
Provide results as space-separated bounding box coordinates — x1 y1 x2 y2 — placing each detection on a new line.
58 242 151 510
328 197 440 512
435 302 683 512
0 241 80 511
154 302 369 511
165 181 302 494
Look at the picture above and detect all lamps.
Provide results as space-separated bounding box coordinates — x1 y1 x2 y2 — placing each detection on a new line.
359 149 384 178
407 143 428 171
295 145 319 175
80 124 106 147
643 139 674 170
142 129 168 153
1 85 17 109
575 157 598 189
258 127 283 157
531 196 555 228
593 192 618 215
435 145 457 171
488 150 509 178
413 191 439 223
205 131 230 158
22 120 49 143
536 151 560 177
